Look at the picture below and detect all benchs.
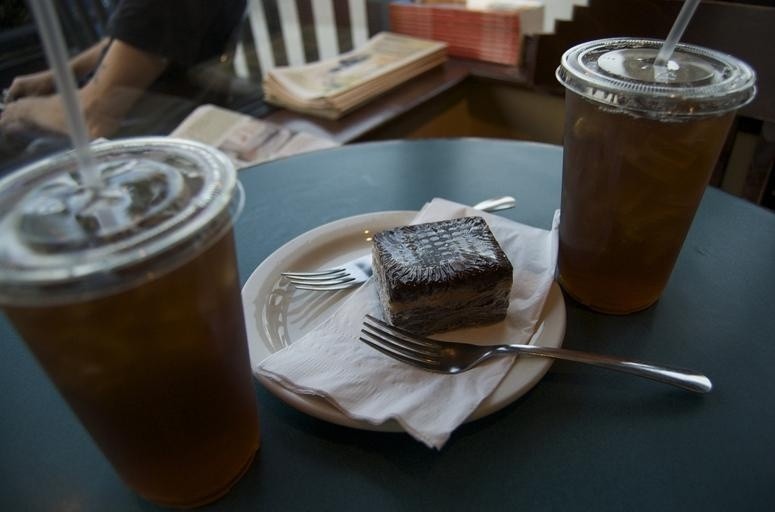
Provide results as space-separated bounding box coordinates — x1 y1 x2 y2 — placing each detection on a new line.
231 2 478 146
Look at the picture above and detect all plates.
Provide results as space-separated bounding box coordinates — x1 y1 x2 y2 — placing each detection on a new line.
242 207 568 433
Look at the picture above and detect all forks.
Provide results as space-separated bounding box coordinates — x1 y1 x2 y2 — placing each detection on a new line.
357 315 714 397
280 191 517 292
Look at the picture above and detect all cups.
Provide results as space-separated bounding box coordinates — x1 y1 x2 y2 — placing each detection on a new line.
1 135 262 510
552 38 759 313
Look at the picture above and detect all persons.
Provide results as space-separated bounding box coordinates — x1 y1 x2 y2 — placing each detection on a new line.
0 0 254 170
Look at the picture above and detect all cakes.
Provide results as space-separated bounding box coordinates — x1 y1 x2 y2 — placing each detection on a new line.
372 217 514 337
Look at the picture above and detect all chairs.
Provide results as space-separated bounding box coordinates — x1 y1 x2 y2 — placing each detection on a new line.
709 114 775 212
